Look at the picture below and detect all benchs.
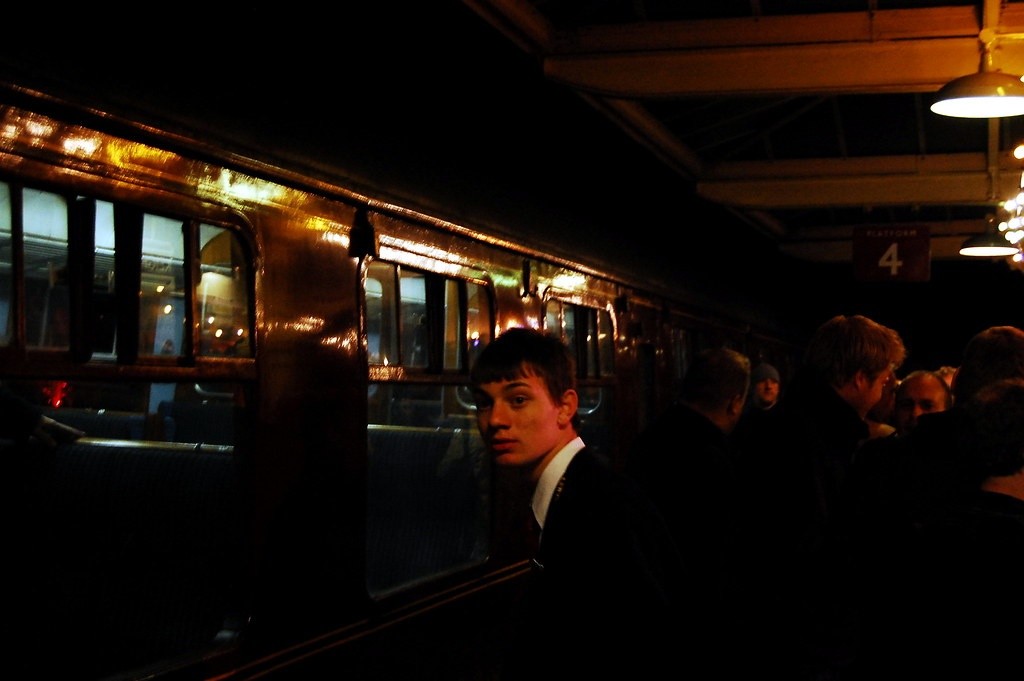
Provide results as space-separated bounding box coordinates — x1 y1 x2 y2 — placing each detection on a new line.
0 379 494 651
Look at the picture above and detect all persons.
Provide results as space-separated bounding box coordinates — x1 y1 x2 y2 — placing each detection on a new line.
625 313 1024 681
469 327 654 681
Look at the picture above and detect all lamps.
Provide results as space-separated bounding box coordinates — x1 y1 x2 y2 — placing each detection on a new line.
928 36 1024 119
958 212 1020 256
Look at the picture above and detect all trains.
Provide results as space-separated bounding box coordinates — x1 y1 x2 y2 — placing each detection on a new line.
0 75 806 681
